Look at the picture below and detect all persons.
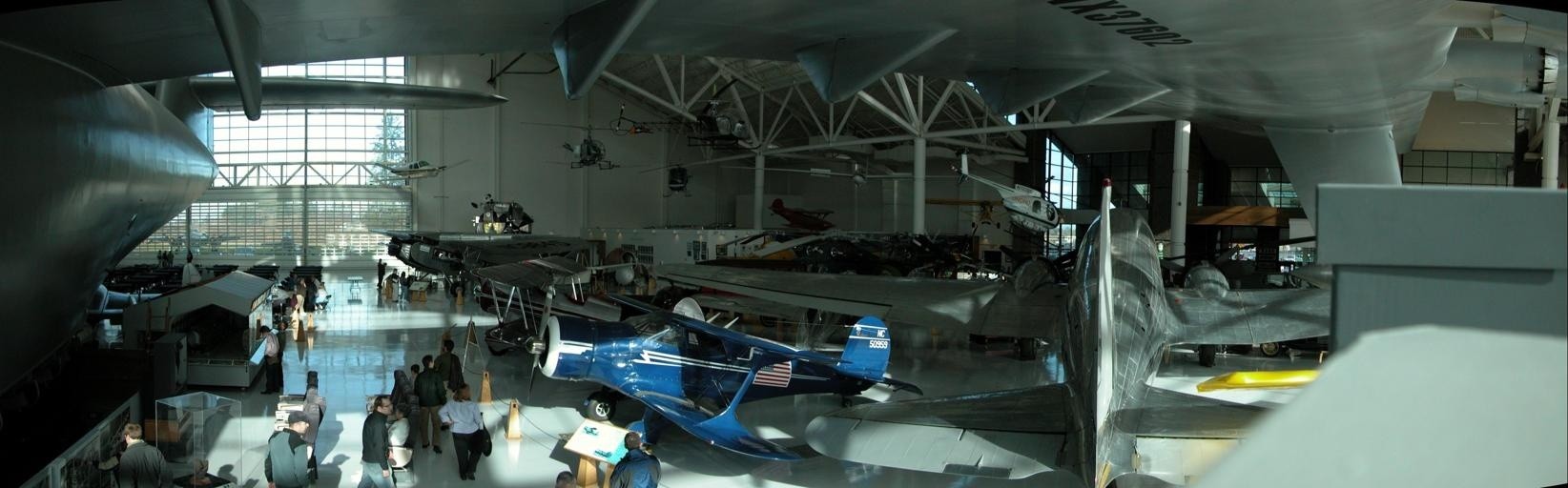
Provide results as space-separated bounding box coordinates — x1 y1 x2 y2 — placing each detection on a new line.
259 321 288 395
116 422 174 487
409 339 463 453
437 383 486 481
553 469 575 487
289 273 327 327
357 396 416 488
264 410 319 488
375 259 407 302
167 250 174 267
608 429 660 488
186 250 193 263
155 250 162 267
161 250 167 266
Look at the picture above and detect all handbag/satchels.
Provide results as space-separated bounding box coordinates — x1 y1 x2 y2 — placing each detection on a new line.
478 426 493 458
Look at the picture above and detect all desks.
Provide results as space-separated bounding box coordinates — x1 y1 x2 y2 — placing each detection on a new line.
348 276 363 292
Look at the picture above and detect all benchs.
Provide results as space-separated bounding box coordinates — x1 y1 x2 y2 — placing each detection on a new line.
103 263 322 294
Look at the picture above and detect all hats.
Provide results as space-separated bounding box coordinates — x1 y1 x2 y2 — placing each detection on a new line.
624 431 643 450
286 409 310 423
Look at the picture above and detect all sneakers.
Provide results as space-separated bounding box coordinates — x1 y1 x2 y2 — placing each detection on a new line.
459 474 476 482
260 383 284 395
422 424 449 455
